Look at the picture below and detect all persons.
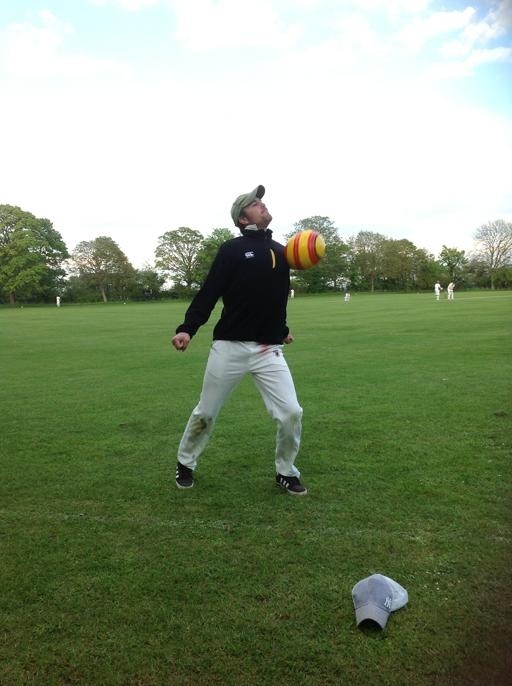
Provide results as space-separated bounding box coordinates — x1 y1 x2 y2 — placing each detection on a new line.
435 279 443 300
289 287 295 298
447 279 456 299
55 293 61 308
343 290 351 304
170 181 309 496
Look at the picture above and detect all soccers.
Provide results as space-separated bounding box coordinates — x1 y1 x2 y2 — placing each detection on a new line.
284 229 325 270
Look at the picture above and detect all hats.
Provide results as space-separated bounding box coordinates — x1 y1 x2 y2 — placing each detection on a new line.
230 184 266 227
353 573 393 630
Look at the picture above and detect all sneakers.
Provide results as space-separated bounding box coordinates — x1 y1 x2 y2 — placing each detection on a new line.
175 460 195 489
274 471 307 495
